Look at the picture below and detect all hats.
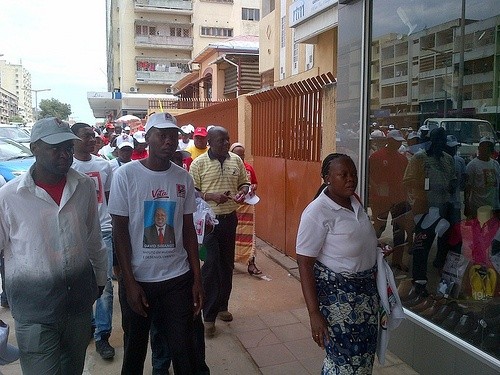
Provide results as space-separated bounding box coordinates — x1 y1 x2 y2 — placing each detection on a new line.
446 135 462 147
479 136 494 145
133 131 147 143
207 126 214 132
180 125 191 135
116 135 134 149
419 124 430 130
125 127 130 131
188 124 194 133
194 127 207 137
230 143 243 153
371 121 378 127
30 117 82 144
371 130 386 139
106 124 114 130
407 131 420 139
387 129 406 142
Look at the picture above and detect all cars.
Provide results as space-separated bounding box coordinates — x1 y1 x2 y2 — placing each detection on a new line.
0 136 37 183
0 124 32 149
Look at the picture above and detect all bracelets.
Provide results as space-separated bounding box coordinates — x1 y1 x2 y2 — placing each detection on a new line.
202 192 207 202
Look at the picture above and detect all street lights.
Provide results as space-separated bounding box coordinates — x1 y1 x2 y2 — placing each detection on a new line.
21 87 52 122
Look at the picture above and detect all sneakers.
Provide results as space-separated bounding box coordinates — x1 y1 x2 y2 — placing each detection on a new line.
95 333 115 359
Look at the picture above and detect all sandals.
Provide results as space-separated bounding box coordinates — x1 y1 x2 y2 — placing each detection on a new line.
248 265 262 275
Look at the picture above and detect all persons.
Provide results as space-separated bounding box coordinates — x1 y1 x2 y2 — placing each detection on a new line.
368 122 500 311
296 153 393 375
0 113 264 375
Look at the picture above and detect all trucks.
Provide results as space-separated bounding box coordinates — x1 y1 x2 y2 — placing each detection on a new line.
423 117 500 166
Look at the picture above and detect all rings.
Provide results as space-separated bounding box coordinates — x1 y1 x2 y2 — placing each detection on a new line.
315 335 319 337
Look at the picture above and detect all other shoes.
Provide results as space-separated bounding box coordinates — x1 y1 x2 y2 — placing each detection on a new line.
390 234 466 300
204 322 215 336
217 309 232 321
